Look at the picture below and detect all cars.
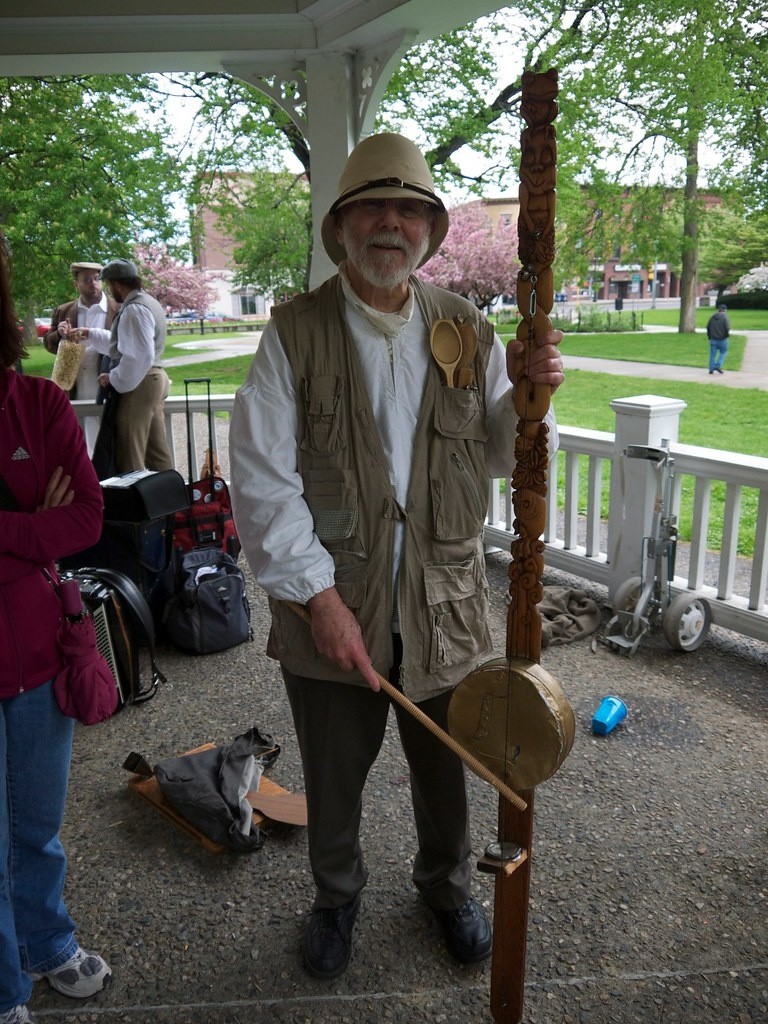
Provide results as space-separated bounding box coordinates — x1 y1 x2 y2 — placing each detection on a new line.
16 317 52 337
165 309 244 323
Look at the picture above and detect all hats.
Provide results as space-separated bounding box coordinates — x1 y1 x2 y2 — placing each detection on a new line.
719 304 728 309
320 132 449 270
69 262 102 277
97 258 139 278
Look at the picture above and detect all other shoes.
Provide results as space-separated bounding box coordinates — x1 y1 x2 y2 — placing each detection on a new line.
715 367 723 373
710 370 715 374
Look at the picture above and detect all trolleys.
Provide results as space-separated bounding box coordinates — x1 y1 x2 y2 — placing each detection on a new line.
602 436 714 657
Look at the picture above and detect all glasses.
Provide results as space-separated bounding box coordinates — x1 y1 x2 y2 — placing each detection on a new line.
354 198 432 218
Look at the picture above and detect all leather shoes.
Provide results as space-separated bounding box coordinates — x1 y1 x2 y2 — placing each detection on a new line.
302 891 361 982
432 896 493 962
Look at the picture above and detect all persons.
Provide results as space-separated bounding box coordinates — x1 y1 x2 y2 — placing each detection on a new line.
707 304 730 374
0 229 176 1024
228 133 565 980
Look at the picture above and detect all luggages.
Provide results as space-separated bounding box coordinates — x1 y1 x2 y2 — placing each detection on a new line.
173 379 243 564
59 566 166 718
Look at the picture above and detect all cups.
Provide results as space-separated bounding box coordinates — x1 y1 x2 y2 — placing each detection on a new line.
592 696 627 735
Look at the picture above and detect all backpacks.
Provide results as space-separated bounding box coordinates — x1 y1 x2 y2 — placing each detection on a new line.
155 548 256 655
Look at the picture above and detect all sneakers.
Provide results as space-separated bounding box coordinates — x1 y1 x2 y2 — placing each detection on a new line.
0 1005 36 1024
22 945 114 998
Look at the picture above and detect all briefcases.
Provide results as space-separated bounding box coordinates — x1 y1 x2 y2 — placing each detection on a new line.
96 467 191 522
